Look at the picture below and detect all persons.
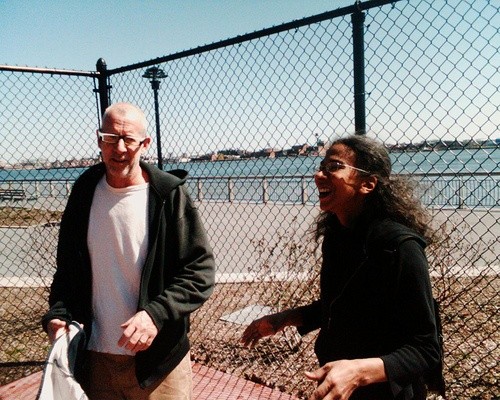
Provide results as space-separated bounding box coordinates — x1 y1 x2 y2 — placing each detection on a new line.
38 100 219 399
241 135 448 400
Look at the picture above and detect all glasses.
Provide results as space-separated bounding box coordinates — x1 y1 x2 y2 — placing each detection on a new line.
316 162 371 174
97 131 148 147
